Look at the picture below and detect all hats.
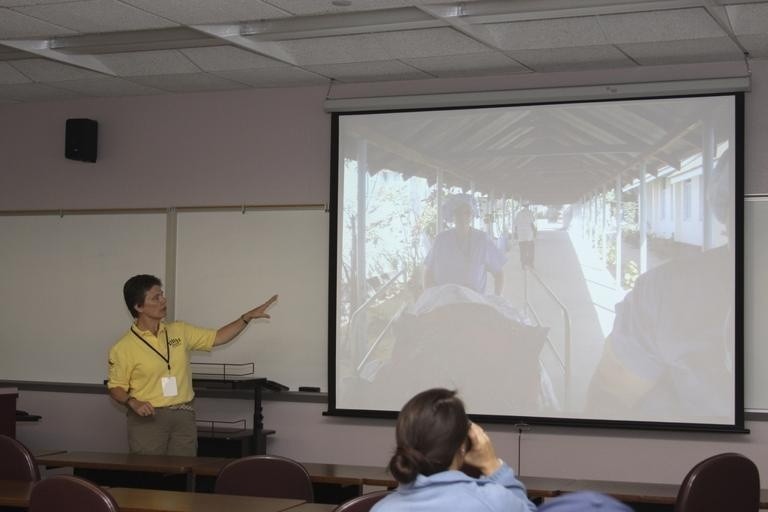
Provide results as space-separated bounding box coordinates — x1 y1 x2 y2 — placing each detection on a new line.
441 193 477 221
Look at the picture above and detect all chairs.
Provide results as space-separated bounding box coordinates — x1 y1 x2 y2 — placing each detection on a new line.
213 454 314 502
673 452 760 511
331 490 393 511
0 433 41 482
29 473 120 511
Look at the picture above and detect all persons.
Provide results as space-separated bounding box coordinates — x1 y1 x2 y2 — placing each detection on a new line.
512 199 539 272
421 194 508 296
585 147 736 425
367 387 538 512
106 274 278 458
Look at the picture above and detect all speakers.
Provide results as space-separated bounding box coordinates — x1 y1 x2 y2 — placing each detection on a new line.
64 118 98 163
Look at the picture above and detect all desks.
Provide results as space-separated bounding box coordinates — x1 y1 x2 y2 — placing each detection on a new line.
0 478 307 511
29 449 68 464
364 468 576 505
281 501 341 511
33 451 388 496
558 479 768 508
